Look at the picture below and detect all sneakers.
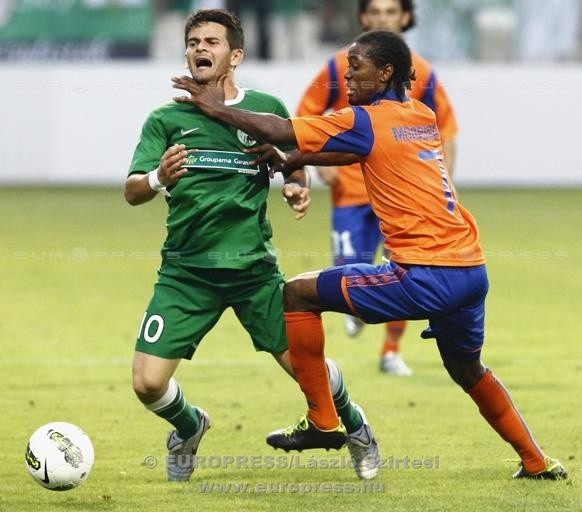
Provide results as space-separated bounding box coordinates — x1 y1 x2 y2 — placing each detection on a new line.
267 417 348 450
513 459 567 480
344 314 362 336
340 403 379 480
380 351 411 377
166 407 209 481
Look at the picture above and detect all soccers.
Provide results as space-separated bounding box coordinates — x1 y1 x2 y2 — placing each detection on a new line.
24 421 93 492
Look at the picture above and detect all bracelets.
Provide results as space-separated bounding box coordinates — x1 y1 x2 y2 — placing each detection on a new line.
149 169 167 194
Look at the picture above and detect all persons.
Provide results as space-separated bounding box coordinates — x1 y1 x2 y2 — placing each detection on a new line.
169 28 571 482
293 0 458 377
121 8 385 483
